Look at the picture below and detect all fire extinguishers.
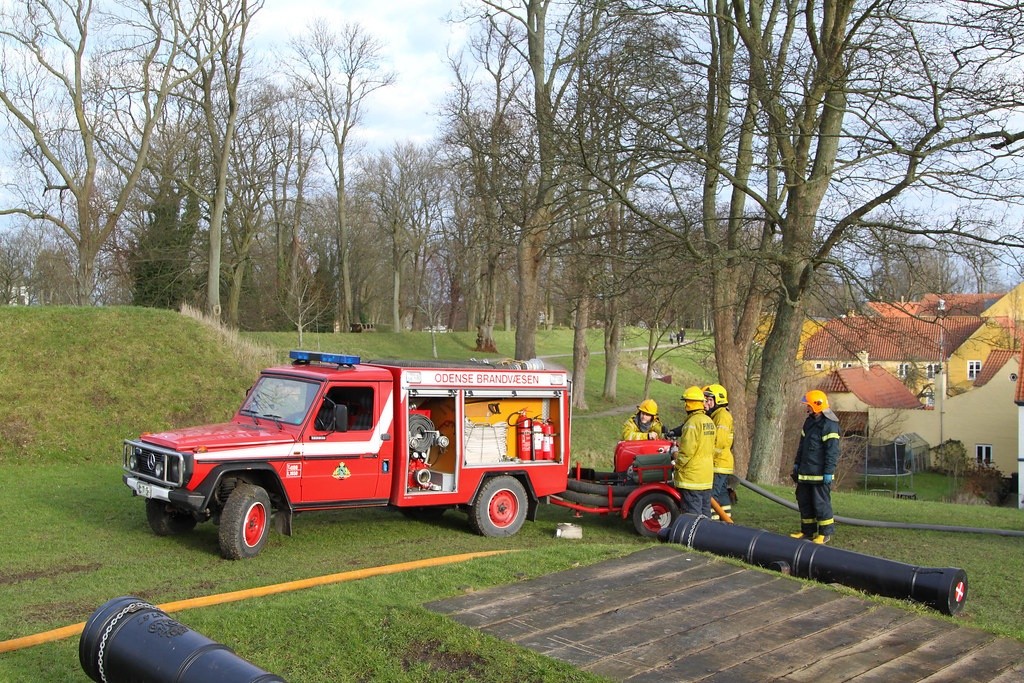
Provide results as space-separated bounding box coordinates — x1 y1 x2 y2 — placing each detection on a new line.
507 407 558 461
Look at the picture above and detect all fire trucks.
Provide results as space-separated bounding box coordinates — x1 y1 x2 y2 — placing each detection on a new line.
122 349 574 560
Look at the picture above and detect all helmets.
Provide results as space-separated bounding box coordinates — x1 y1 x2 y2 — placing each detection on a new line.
680 385 706 401
704 383 729 406
637 398 657 415
801 390 829 413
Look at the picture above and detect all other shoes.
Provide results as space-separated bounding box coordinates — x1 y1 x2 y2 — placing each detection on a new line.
813 535 831 544
790 532 814 539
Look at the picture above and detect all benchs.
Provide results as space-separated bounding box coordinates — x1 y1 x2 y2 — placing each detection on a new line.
351 323 376 333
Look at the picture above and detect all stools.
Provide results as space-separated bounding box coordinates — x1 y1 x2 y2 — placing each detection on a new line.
897 491 917 500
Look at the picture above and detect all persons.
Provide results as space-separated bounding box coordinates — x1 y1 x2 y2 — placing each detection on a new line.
668 384 734 526
621 399 667 441
790 388 840 544
673 385 717 520
670 328 686 346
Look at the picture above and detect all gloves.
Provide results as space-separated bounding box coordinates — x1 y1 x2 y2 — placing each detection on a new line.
794 465 798 471
823 474 832 484
